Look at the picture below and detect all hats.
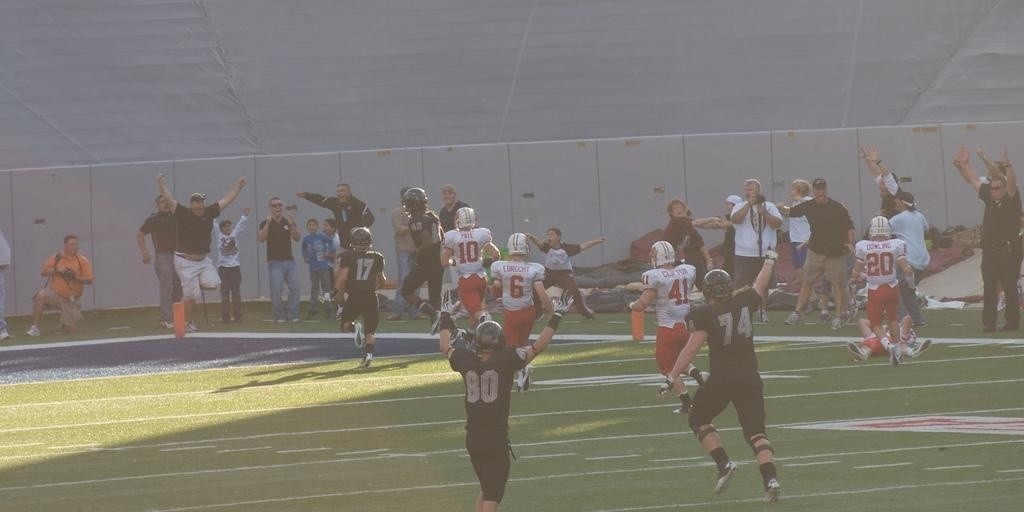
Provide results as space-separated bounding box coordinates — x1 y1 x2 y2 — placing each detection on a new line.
726 195 742 205
190 193 207 201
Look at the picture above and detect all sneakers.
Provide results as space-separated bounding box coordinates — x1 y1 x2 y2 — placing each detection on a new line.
27 325 40 336
714 460 736 494
672 370 710 413
161 319 198 333
362 352 373 367
354 321 363 349
517 365 533 393
785 298 859 329
430 310 441 335
848 339 931 367
763 479 780 503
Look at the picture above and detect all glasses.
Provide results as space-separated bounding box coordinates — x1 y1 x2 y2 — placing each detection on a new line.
273 203 283 207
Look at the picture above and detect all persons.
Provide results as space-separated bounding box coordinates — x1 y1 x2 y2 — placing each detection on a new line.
26 235 93 336
857 145 902 219
136 174 608 401
0 230 11 340
656 241 779 503
628 178 931 409
437 291 575 510
953 145 1022 331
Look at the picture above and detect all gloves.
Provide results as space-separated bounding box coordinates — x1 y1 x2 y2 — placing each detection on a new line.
441 289 460 315
657 377 673 397
551 295 574 315
765 245 778 266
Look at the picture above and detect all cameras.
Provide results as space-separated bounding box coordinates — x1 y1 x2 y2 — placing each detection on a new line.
61 270 74 282
756 194 766 204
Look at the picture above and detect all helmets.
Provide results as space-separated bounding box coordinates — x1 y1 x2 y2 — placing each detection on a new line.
474 321 502 351
401 188 428 217
650 240 675 268
509 232 530 255
349 228 372 249
702 269 733 300
869 216 891 239
455 207 475 230
